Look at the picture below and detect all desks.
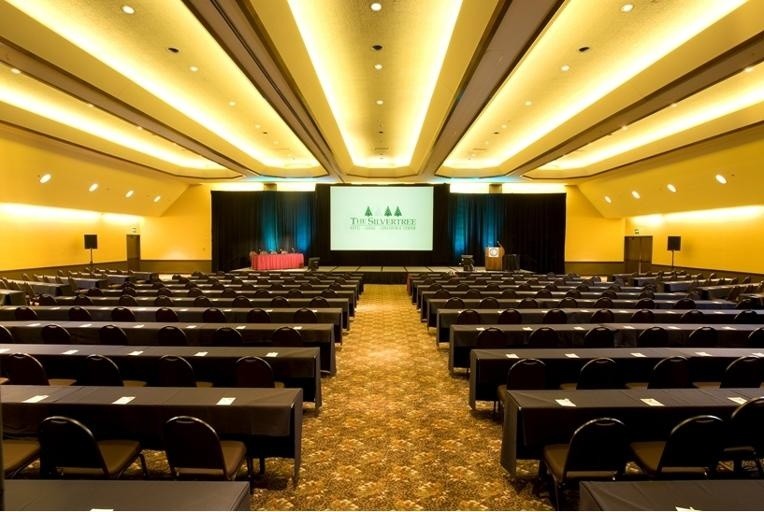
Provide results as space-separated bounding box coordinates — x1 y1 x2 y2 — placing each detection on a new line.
0 384 302 485
579 477 764 511
252 252 304 271
0 479 249 509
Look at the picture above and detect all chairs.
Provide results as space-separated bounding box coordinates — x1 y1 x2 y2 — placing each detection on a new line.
716 397 764 481
536 419 628 508
630 414 723 482
38 416 147 482
1 438 41 477
249 249 288 262
162 418 242 481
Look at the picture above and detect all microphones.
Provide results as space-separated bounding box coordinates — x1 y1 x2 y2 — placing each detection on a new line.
496 241 503 247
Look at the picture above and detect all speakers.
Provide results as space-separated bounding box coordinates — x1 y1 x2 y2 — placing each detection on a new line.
84 234 98 249
668 236 681 250
